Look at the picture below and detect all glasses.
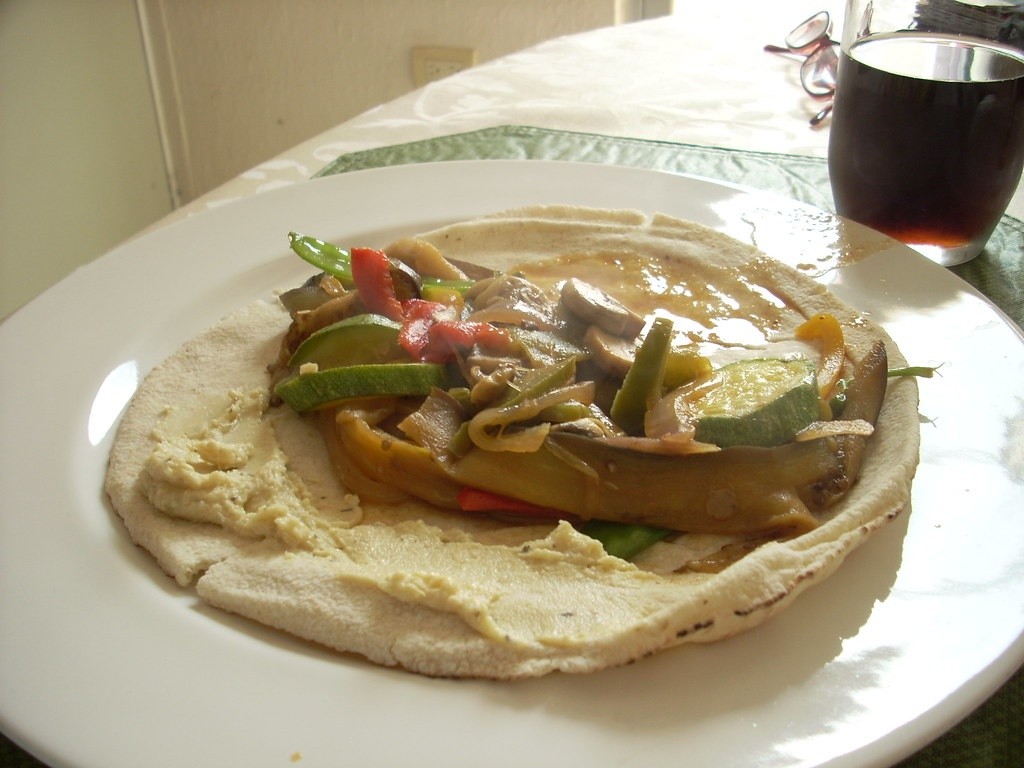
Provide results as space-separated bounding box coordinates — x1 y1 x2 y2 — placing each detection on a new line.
765 11 840 125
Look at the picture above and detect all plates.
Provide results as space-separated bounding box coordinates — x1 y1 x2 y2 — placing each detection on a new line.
0 160 1024 768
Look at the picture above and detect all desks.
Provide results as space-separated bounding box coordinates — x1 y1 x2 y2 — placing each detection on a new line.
0 1 1024 768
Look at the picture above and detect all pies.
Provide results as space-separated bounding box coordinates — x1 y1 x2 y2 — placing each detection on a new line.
106 204 920 680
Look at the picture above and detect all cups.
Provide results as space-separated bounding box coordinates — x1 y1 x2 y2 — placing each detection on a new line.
827 0 1024 267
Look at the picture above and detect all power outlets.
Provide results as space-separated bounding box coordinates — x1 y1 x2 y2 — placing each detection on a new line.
411 45 476 88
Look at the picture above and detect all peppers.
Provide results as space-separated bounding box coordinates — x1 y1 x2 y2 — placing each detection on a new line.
288 232 476 290
456 487 569 518
